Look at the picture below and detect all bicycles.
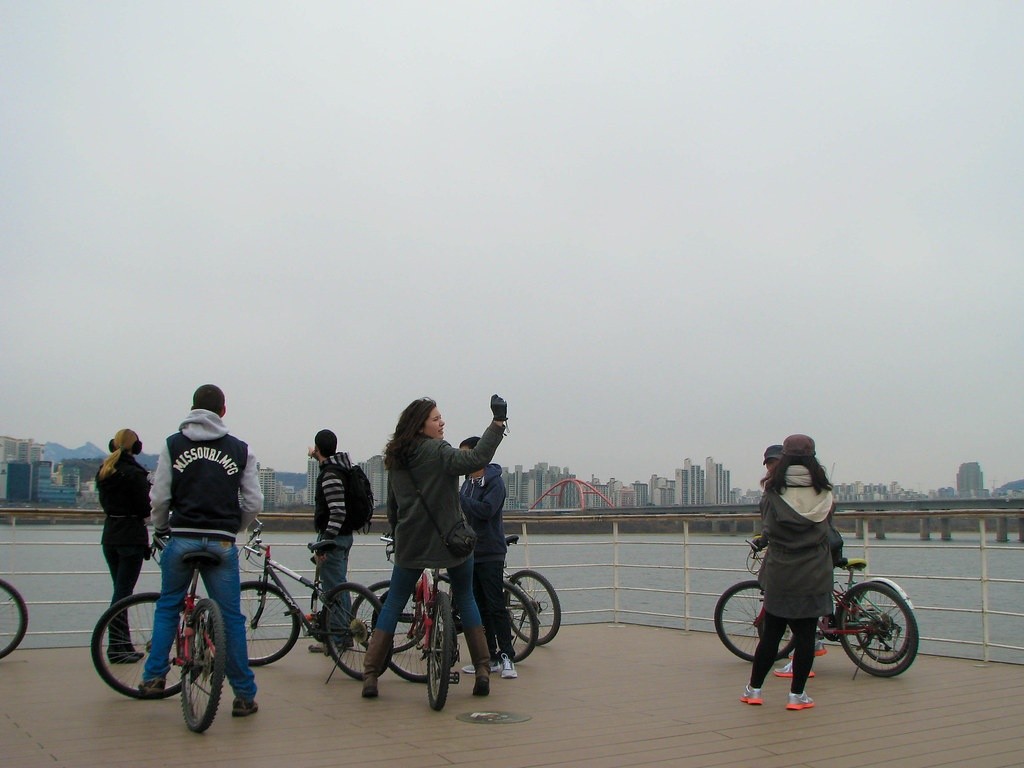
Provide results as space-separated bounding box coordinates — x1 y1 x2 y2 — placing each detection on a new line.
349 531 564 711
237 515 394 686
0 578 27 659
91 533 226 734
713 539 920 683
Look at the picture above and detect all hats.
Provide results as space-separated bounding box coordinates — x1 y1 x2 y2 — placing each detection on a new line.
763 445 782 465
781 434 816 456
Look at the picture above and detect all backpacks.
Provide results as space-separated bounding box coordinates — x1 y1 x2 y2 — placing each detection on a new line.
319 464 376 533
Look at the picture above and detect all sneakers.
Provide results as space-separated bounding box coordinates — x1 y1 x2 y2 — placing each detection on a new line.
773 640 827 678
138 679 166 695
785 691 815 710
232 699 259 718
462 660 500 674
501 652 518 679
739 683 764 705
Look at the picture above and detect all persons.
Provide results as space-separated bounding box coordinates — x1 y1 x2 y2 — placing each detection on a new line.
96 429 152 663
763 445 827 677
362 394 508 697
740 433 836 710
139 384 263 716
308 429 354 653
460 436 516 678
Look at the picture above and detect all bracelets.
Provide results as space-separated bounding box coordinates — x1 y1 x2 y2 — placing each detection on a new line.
311 450 315 459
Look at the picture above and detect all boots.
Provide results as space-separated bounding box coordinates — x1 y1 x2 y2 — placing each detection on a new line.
107 631 144 663
463 624 491 695
361 628 394 697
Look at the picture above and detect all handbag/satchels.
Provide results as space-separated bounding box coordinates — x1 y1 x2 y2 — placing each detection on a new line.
445 520 476 558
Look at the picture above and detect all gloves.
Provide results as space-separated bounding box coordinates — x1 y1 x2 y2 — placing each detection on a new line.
154 527 171 551
490 394 508 421
752 533 768 552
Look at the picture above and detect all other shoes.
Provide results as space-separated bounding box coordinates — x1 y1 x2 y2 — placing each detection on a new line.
308 638 355 654
